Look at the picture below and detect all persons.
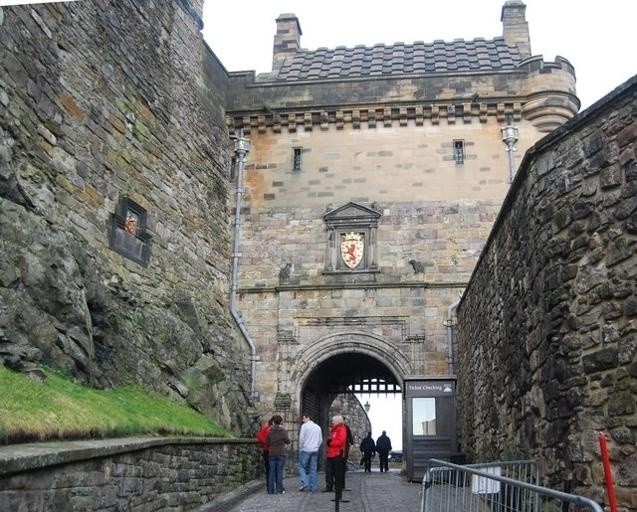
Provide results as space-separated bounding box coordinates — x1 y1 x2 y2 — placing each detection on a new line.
257 422 270 471
265 413 289 494
342 417 354 458
325 414 346 494
360 430 375 472
375 430 392 470
296 412 324 491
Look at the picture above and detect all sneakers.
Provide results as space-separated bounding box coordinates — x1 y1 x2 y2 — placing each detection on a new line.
321 488 331 492
299 484 307 491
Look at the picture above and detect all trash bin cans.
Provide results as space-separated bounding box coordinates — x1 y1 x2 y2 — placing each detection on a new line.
450 452 467 487
